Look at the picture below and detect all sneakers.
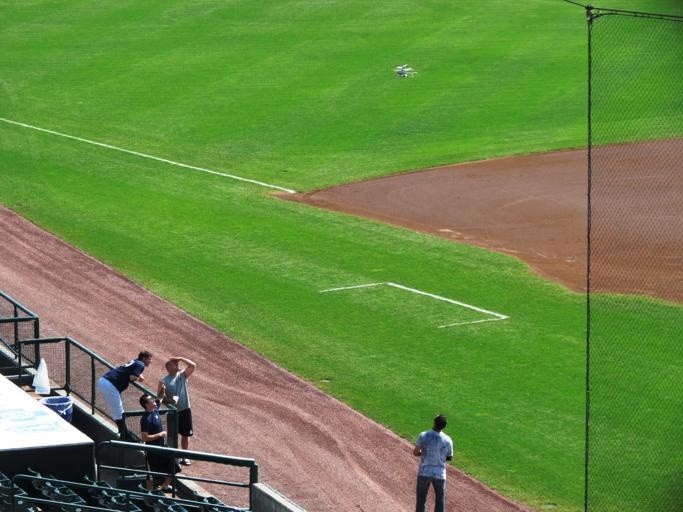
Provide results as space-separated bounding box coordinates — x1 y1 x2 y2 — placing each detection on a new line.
176 457 192 466
161 485 179 493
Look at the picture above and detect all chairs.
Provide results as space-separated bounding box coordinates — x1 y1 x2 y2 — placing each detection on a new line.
0 467 234 512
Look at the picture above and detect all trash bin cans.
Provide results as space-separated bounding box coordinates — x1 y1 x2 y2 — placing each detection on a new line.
41 396 73 423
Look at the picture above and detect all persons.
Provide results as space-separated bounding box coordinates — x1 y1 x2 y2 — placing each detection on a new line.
136 384 178 497
409 413 454 511
155 356 197 467
97 350 152 439
